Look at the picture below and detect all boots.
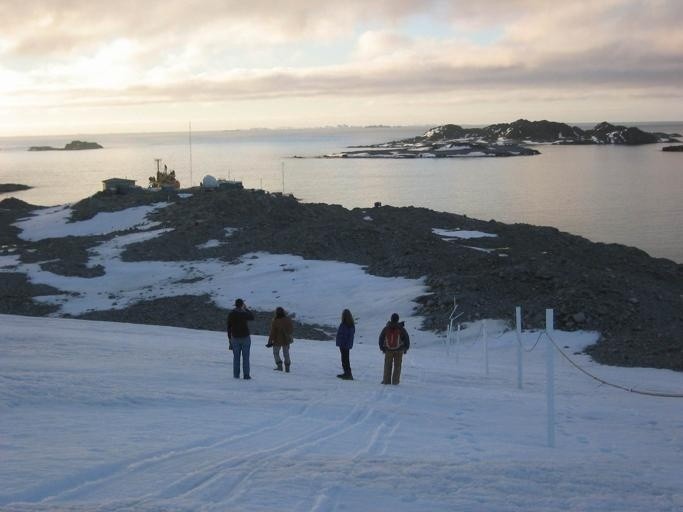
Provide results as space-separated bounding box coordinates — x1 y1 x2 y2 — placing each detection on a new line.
274 361 283 371
336 367 353 379
285 363 290 373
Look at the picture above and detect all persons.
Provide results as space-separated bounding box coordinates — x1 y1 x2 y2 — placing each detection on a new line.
227 298 254 379
336 309 355 379
379 313 410 385
268 307 294 372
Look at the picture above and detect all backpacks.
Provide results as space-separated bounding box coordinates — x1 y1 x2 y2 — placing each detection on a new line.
382 327 403 350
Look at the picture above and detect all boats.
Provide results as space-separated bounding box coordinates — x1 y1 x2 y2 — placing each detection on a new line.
149 155 181 192
